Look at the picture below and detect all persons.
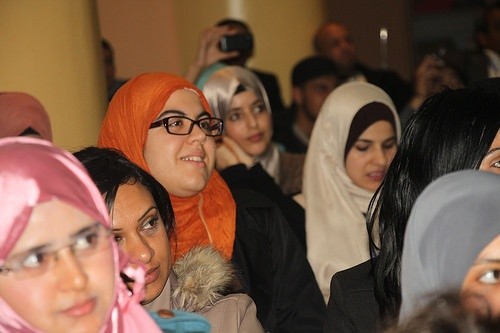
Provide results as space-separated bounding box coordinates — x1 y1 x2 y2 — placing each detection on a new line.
377 288 500 333
71 145 267 333
0 136 213 333
0 90 54 141
398 170 500 316
321 86 500 333
184 15 500 308
99 38 130 101
97 72 326 333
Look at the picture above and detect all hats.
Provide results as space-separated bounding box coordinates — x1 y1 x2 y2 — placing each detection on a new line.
292 57 334 88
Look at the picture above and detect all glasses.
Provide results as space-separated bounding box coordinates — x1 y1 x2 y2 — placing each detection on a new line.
0 228 115 277
148 115 223 136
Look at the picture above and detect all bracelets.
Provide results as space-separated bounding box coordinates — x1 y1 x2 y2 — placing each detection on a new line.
192 61 203 67
246 158 258 170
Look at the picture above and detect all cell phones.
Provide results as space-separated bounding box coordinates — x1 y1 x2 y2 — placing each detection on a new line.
220 32 252 51
